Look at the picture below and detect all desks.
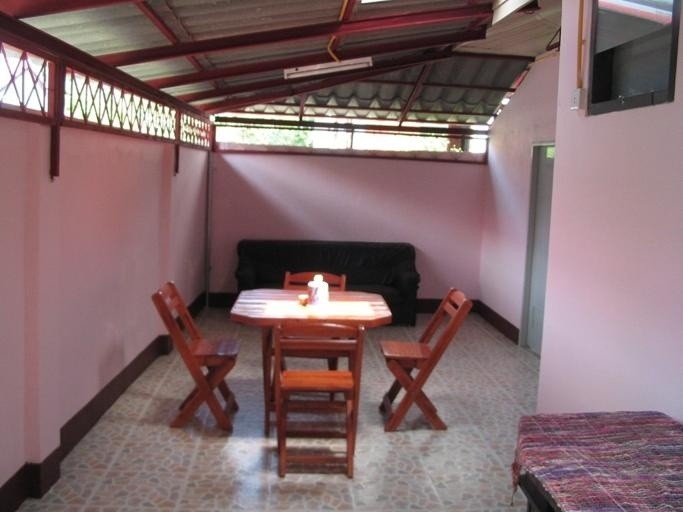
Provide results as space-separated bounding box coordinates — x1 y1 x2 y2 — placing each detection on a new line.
228 289 394 436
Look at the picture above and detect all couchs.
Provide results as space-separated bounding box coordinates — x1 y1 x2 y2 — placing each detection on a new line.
234 239 421 327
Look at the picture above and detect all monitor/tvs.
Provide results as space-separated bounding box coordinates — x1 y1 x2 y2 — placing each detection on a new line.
585 0 681 116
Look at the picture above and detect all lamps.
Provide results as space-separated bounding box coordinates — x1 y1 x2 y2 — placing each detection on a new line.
283 57 373 80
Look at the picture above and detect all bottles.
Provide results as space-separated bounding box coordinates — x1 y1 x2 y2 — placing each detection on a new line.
307 274 329 306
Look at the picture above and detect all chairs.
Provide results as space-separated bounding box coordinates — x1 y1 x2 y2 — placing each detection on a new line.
151 280 241 432
381 287 475 433
273 270 348 402
275 320 365 477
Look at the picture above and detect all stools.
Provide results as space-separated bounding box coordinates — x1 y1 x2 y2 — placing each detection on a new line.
511 409 683 512
